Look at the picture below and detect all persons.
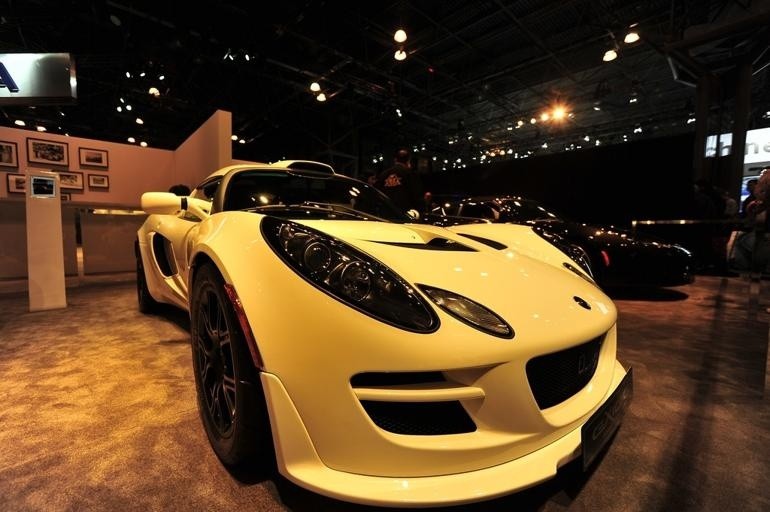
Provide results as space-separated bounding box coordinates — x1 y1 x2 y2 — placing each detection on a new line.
423 192 434 213
353 172 378 217
694 165 770 273
375 149 426 224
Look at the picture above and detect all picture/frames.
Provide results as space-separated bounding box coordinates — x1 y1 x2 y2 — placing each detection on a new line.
0 137 109 201
